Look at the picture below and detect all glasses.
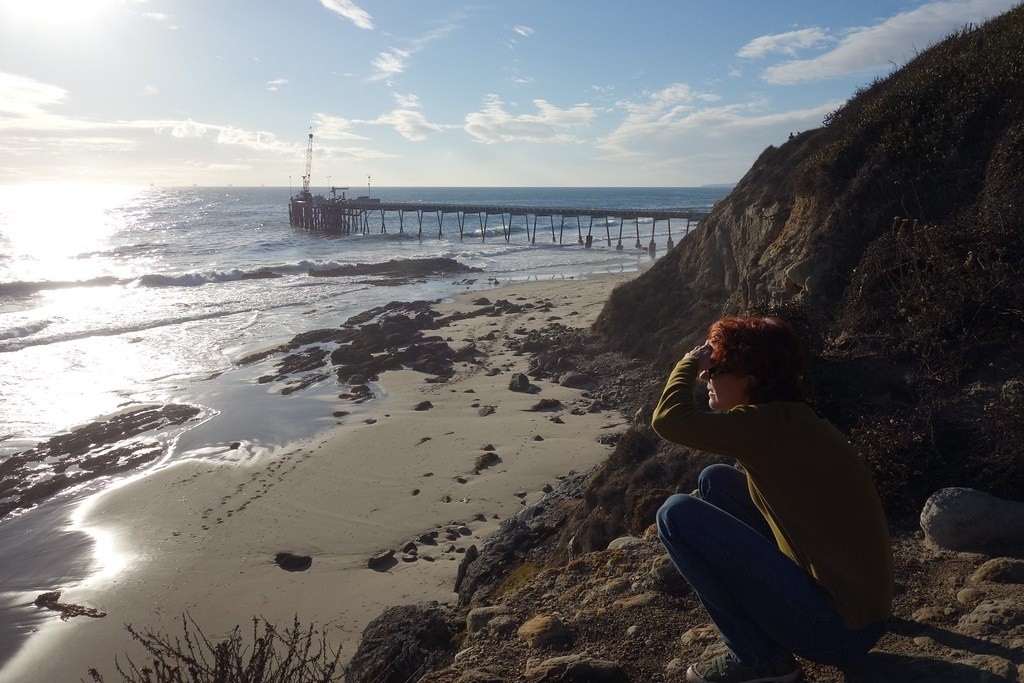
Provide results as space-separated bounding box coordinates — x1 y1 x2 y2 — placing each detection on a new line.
707 364 745 379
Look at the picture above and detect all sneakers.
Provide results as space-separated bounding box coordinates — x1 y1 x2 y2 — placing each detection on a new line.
685 653 800 683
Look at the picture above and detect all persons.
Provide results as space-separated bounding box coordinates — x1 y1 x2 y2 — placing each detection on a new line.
651 312 895 683
